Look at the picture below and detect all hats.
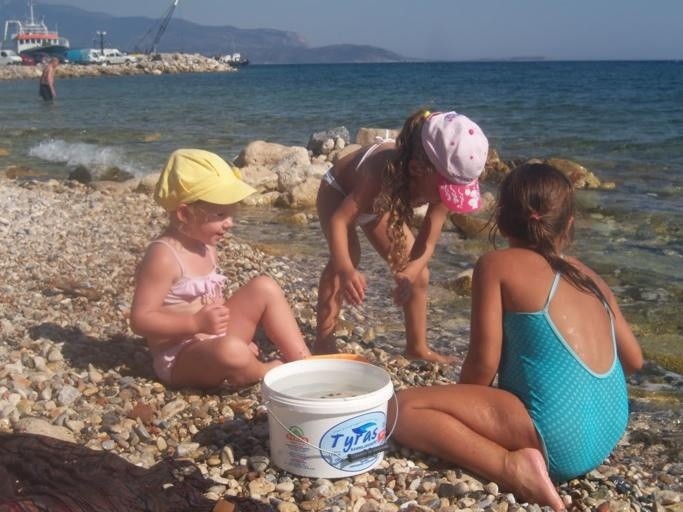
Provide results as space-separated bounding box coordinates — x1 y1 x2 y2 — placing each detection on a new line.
421 109 489 215
154 148 257 212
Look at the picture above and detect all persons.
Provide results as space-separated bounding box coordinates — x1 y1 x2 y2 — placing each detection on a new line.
39 56 59 103
387 164 644 512
129 148 313 388
313 111 489 366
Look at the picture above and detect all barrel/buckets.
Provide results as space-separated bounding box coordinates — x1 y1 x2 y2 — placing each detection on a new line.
263 359 399 479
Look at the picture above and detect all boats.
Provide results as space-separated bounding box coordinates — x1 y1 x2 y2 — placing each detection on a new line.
218 53 248 66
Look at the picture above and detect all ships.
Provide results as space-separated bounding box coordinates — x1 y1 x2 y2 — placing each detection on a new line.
3 0 69 55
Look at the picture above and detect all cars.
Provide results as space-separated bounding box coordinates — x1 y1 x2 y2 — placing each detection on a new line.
0 44 136 66
